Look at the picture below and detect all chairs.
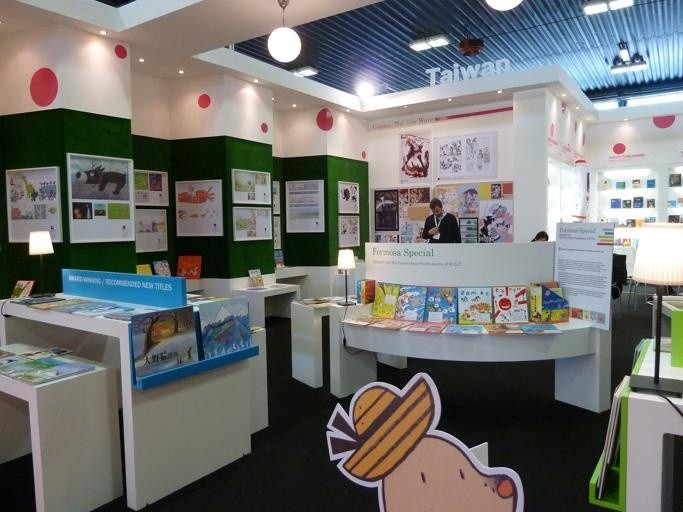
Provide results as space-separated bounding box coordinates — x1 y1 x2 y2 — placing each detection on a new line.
612 253 627 317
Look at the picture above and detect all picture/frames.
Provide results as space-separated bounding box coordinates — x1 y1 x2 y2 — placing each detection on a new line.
285 179 325 234
375 189 399 231
65 152 170 253
231 168 282 249
337 180 361 248
4 166 64 243
174 179 224 238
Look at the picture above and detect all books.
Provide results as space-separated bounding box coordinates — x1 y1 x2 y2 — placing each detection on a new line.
153 259 171 276
248 267 264 286
137 264 152 278
595 374 632 502
0 280 253 386
340 278 571 337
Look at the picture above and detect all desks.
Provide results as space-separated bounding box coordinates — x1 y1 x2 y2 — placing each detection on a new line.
232 283 302 328
1 289 251 511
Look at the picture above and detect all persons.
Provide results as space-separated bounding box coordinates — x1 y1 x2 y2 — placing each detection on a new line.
402 133 430 178
531 230 549 241
422 199 462 244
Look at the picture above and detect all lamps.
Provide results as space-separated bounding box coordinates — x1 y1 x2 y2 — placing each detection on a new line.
630 223 682 385
27 231 55 298
610 40 649 74
336 249 357 307
